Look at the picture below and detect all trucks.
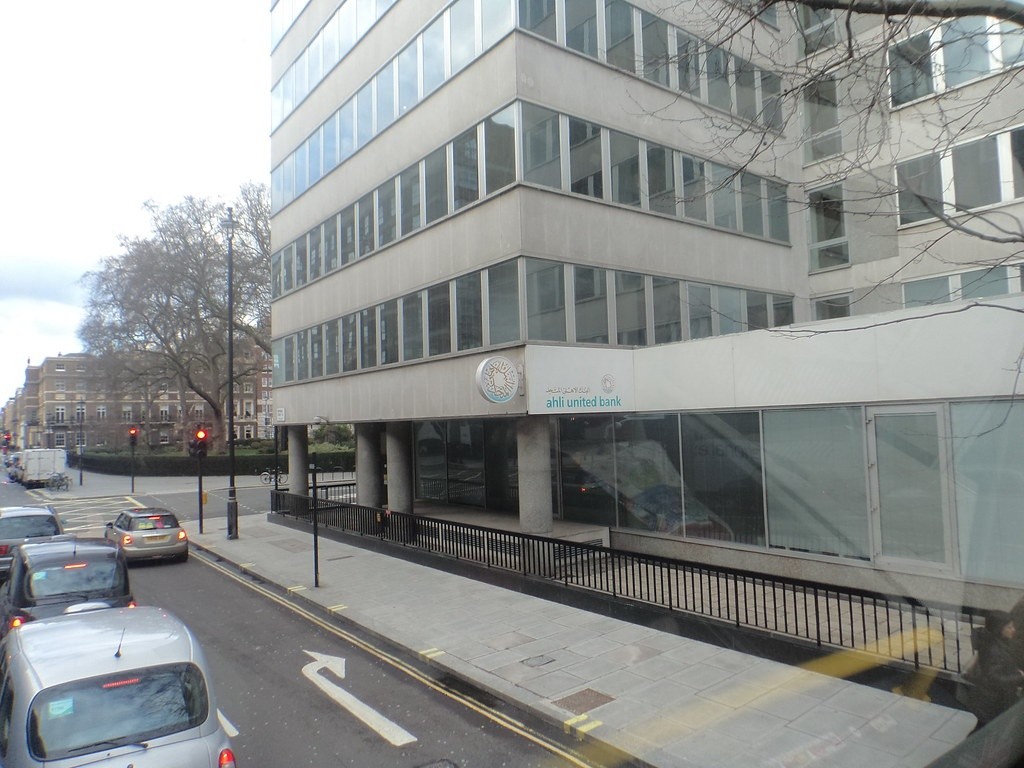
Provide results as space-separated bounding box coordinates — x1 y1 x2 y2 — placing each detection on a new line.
15 448 66 489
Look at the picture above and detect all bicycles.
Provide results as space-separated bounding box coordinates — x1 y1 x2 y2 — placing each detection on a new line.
44 472 72 492
260 466 288 484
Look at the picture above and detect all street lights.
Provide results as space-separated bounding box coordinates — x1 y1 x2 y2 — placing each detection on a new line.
221 207 240 539
77 397 85 486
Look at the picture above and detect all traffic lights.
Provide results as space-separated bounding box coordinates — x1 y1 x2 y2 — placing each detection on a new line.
196 430 207 459
129 428 137 446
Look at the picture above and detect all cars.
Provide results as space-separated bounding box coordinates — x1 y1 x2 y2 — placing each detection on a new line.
105 508 188 566
4 452 21 466
0 603 236 767
0 535 133 608
0 483 64 580
